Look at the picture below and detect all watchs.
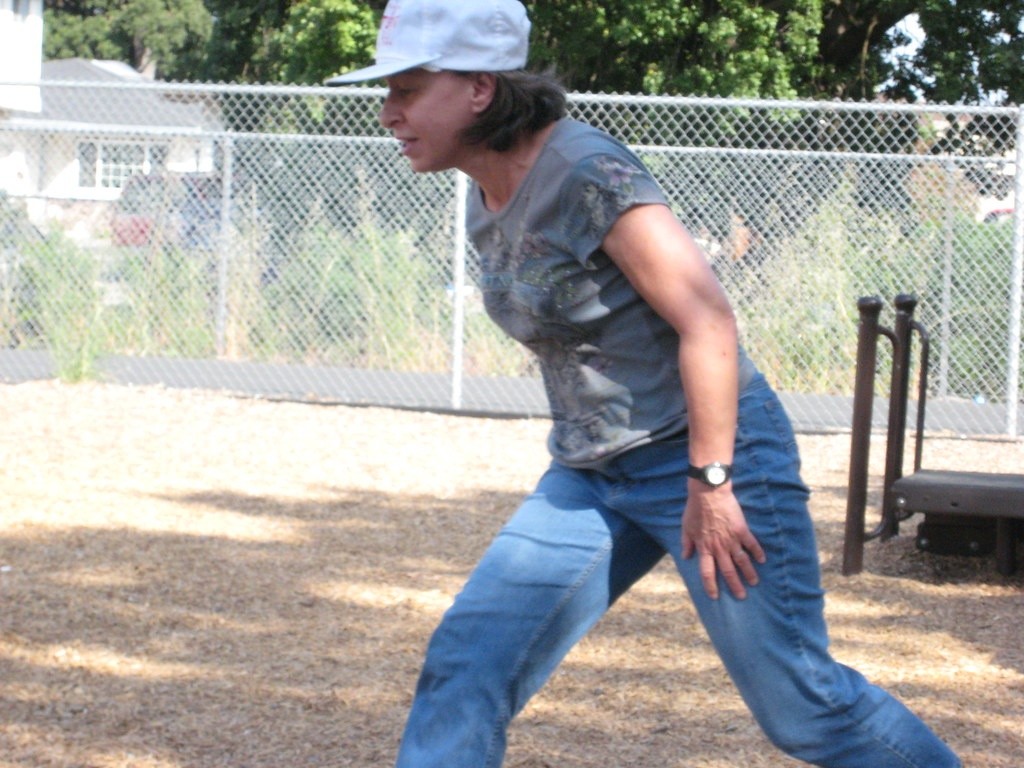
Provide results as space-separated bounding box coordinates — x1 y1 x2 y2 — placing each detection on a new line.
689 461 734 487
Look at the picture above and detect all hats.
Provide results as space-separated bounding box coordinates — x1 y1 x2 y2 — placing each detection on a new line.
323 0 531 87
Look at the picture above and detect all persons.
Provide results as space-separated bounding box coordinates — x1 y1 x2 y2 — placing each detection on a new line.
327 1 964 767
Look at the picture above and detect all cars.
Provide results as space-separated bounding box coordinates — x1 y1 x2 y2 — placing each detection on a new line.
108 169 228 251
